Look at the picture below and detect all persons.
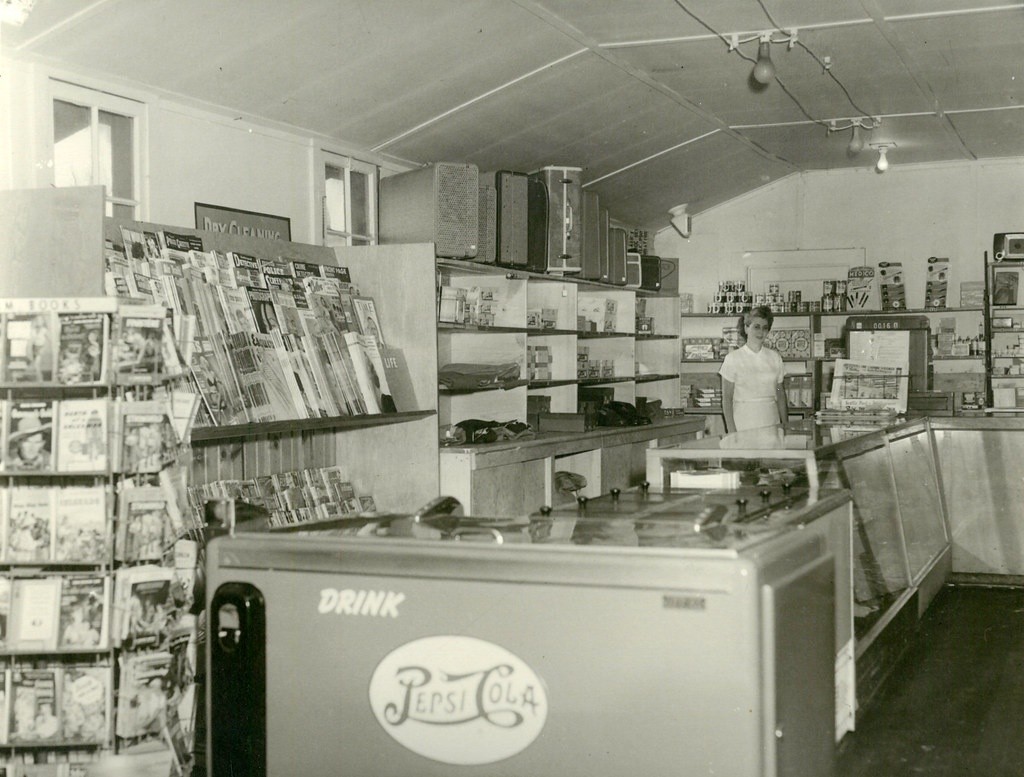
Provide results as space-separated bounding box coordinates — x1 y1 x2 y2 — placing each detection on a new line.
718 307 788 434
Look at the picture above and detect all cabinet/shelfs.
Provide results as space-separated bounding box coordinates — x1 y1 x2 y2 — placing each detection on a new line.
0 185 1024 772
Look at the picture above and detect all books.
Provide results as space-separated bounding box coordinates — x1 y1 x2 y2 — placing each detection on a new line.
0 225 420 777
